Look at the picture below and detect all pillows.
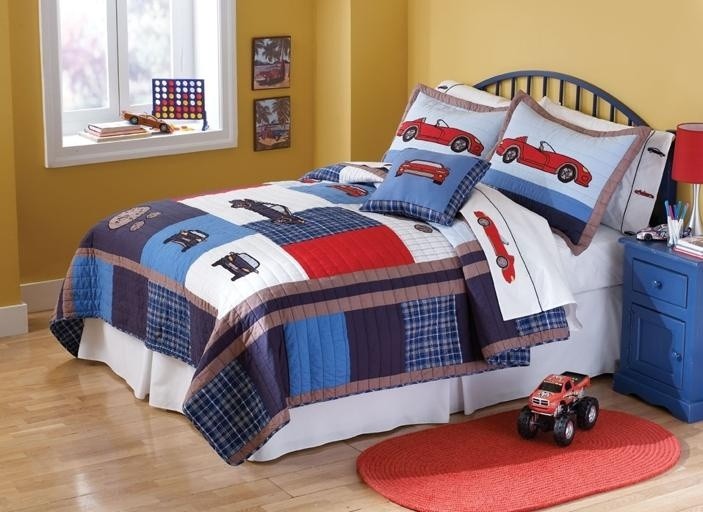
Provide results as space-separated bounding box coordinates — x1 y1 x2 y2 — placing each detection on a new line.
540 96 674 234
381 83 510 165
479 89 651 254
435 79 511 107
361 147 492 226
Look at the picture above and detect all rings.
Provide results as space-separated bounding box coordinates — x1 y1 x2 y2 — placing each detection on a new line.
665 201 689 246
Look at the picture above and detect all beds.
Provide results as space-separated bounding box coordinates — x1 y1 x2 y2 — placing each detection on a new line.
86 68 679 463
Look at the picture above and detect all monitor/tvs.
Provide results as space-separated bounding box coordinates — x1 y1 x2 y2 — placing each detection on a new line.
78 121 152 143
674 235 703 259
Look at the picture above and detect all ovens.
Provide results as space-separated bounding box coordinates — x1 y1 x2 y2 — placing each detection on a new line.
86 68 679 463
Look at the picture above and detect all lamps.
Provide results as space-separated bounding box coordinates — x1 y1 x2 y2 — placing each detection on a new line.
670 121 703 237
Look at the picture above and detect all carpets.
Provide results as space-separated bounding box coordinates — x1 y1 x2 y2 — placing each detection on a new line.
354 404 679 510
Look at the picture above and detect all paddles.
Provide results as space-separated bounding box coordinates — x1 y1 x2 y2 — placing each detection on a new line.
354 404 679 510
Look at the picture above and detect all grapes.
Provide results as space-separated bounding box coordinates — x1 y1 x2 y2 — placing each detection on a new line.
618 230 703 424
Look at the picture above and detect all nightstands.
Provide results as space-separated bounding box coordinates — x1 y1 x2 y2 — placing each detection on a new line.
618 230 703 424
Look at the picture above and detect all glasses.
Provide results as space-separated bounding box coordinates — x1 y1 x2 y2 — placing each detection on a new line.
670 121 703 237
